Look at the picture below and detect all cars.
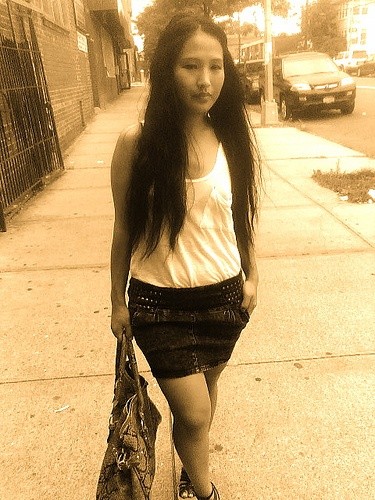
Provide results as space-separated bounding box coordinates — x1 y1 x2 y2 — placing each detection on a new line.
236 57 269 105
357 61 375 78
258 50 358 122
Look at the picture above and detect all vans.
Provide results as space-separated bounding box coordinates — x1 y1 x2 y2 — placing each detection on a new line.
332 50 368 74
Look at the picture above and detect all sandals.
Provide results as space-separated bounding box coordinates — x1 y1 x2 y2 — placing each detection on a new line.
179 468 196 499
193 482 220 500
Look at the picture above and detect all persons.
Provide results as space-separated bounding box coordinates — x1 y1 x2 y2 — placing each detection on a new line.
110 14 261 500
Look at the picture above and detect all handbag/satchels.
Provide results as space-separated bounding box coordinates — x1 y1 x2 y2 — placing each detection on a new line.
95 331 163 500
